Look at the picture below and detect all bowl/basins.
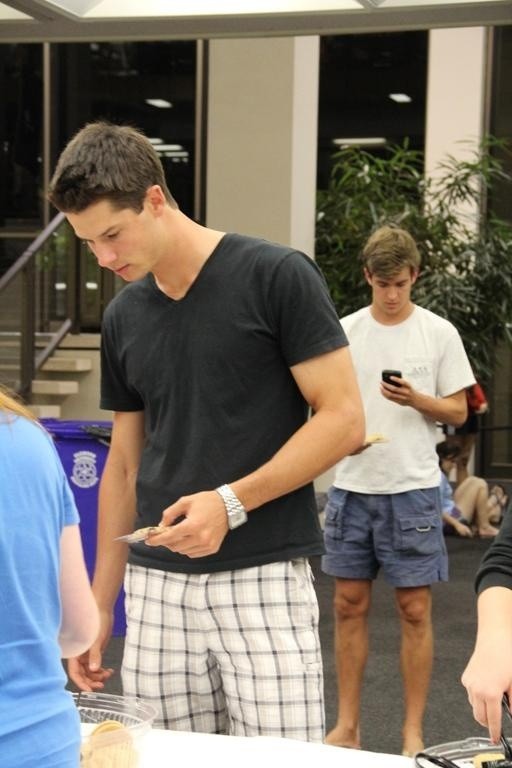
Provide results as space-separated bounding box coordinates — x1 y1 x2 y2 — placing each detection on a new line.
71 691 159 768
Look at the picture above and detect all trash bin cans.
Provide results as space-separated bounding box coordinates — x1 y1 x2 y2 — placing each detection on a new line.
39 418 127 638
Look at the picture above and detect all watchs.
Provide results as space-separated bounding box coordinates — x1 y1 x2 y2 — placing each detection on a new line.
214 483 250 535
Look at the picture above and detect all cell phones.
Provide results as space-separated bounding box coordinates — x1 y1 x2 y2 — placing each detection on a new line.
382 369 402 388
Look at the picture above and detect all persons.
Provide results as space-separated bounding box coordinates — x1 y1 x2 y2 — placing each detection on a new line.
462 497 511 747
441 380 490 467
484 479 509 526
45 117 370 744
319 223 479 759
435 439 502 539
2 381 103 767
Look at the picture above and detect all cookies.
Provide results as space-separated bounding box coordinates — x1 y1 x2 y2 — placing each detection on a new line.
81 721 138 768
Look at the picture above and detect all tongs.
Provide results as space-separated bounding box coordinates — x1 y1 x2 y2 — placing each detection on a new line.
499 692 512 768
